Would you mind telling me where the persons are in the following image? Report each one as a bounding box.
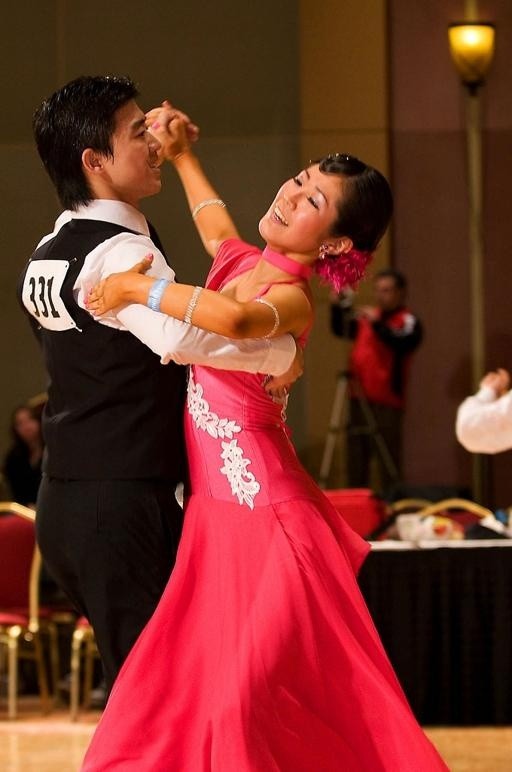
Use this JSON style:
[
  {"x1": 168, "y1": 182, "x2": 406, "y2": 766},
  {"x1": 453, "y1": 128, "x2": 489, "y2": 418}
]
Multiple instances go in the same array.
[
  {"x1": 25, "y1": 75, "x2": 302, "y2": 688},
  {"x1": 454, "y1": 361, "x2": 511, "y2": 454},
  {"x1": 2, "y1": 405, "x2": 47, "y2": 502},
  {"x1": 80, "y1": 94, "x2": 457, "y2": 772},
  {"x1": 329, "y1": 267, "x2": 423, "y2": 493}
]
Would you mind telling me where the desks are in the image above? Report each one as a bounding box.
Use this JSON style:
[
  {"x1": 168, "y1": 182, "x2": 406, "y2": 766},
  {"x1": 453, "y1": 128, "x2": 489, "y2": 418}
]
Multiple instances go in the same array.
[{"x1": 356, "y1": 548, "x2": 512, "y2": 726}]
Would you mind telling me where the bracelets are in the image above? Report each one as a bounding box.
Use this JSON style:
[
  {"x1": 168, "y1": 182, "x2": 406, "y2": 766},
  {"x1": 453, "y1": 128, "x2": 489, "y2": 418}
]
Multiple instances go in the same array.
[{"x1": 147, "y1": 276, "x2": 172, "y2": 311}]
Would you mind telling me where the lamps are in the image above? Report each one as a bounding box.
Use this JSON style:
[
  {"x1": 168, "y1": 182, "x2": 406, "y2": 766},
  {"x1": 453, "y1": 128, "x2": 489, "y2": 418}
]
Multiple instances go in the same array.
[{"x1": 448, "y1": 20, "x2": 496, "y2": 98}]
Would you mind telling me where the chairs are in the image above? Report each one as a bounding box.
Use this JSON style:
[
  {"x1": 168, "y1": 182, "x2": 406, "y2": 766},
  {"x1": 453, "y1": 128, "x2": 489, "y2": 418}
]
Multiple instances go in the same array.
[
  {"x1": 0, "y1": 502, "x2": 105, "y2": 723},
  {"x1": 387, "y1": 498, "x2": 496, "y2": 540}
]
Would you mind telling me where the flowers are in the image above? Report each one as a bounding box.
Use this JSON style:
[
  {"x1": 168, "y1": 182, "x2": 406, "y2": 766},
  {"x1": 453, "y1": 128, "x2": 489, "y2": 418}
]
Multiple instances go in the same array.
[{"x1": 317, "y1": 248, "x2": 375, "y2": 296}]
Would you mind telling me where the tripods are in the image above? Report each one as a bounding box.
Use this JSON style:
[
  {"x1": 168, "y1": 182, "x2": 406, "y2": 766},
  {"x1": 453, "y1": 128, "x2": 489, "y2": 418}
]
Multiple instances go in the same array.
[{"x1": 319, "y1": 338, "x2": 400, "y2": 489}]
